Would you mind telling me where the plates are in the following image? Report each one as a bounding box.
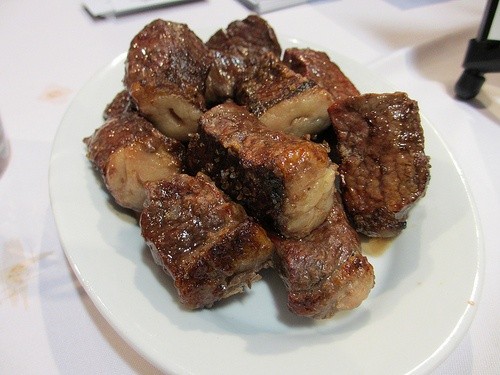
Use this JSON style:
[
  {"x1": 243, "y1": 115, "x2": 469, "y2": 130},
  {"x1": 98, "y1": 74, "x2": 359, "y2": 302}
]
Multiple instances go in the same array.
[{"x1": 46, "y1": 31, "x2": 484, "y2": 375}]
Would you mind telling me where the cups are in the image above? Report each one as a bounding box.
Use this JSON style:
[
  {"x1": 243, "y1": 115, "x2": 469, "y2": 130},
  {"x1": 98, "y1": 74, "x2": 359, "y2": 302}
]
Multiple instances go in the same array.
[{"x1": 0, "y1": 117, "x2": 13, "y2": 176}]
